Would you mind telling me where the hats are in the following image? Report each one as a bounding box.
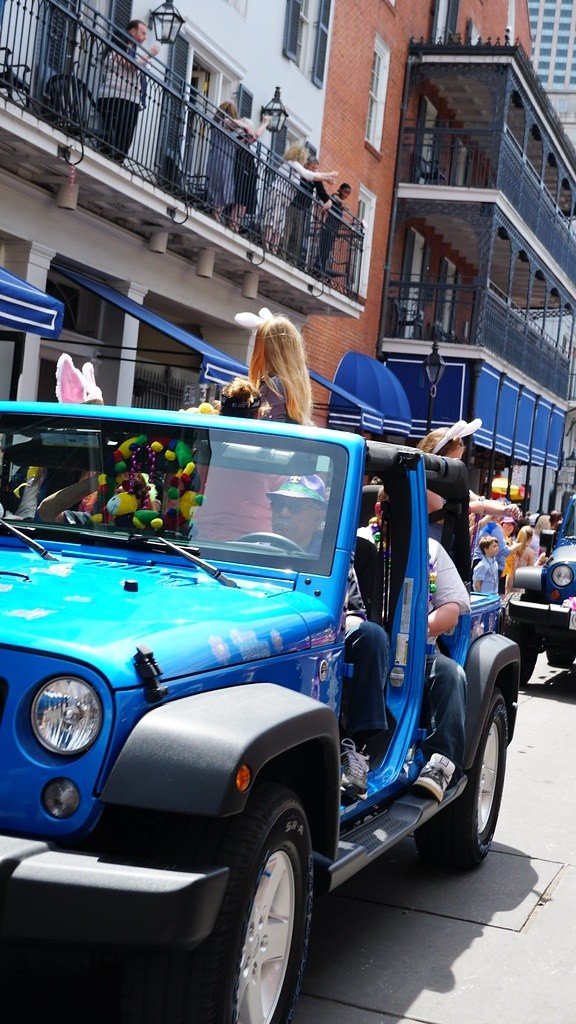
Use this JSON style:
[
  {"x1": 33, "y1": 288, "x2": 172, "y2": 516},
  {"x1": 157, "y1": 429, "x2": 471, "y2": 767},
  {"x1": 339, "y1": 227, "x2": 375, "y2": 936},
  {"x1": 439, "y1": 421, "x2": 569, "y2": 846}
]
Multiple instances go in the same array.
[
  {"x1": 500, "y1": 515, "x2": 517, "y2": 526},
  {"x1": 265, "y1": 472, "x2": 326, "y2": 503}
]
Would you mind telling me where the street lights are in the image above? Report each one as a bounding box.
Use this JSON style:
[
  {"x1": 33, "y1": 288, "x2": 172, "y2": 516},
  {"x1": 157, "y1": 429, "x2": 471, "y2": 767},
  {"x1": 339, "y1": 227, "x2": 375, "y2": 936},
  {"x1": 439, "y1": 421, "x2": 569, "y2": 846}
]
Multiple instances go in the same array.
[{"x1": 423, "y1": 338, "x2": 447, "y2": 434}]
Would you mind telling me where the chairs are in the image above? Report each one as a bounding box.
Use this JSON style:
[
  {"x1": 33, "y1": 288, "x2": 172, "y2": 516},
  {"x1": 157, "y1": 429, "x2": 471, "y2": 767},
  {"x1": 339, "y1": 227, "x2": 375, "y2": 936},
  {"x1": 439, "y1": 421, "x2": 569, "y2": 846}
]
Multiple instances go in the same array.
[
  {"x1": 166, "y1": 154, "x2": 215, "y2": 215},
  {"x1": 325, "y1": 253, "x2": 350, "y2": 285},
  {"x1": 42, "y1": 74, "x2": 115, "y2": 159},
  {"x1": 393, "y1": 297, "x2": 466, "y2": 344},
  {"x1": 225, "y1": 201, "x2": 262, "y2": 243},
  {"x1": 310, "y1": 529, "x2": 378, "y2": 621},
  {"x1": 415, "y1": 153, "x2": 448, "y2": 185},
  {"x1": 0, "y1": 47, "x2": 31, "y2": 110}
]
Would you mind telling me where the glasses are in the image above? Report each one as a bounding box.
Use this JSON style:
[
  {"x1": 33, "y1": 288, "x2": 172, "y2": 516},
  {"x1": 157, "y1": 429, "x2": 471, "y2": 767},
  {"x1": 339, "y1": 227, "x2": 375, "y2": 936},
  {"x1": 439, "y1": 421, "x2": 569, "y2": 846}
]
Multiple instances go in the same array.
[{"x1": 268, "y1": 498, "x2": 306, "y2": 514}]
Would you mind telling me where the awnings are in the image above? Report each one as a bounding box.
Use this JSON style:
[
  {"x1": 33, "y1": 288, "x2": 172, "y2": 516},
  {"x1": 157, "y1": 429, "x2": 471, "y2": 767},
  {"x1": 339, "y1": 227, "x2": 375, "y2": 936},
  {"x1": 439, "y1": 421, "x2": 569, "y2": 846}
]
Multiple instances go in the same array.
[
  {"x1": 0, "y1": 265, "x2": 62, "y2": 339},
  {"x1": 52, "y1": 263, "x2": 249, "y2": 388},
  {"x1": 326, "y1": 350, "x2": 413, "y2": 437},
  {"x1": 306, "y1": 365, "x2": 384, "y2": 433}
]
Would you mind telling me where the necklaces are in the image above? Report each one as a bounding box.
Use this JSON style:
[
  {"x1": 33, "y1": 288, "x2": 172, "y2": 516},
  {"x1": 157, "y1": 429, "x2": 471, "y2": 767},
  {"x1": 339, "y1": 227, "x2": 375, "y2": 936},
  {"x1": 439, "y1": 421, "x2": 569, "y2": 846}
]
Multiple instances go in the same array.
[
  {"x1": 369, "y1": 514, "x2": 436, "y2": 603},
  {"x1": 471, "y1": 496, "x2": 485, "y2": 557}
]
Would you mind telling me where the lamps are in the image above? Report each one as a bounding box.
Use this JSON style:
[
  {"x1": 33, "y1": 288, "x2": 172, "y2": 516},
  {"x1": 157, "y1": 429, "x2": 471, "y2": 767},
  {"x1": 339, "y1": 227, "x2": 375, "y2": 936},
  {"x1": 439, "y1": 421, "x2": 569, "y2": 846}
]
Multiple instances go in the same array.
[
  {"x1": 259, "y1": 86, "x2": 288, "y2": 133},
  {"x1": 149, "y1": 0, "x2": 185, "y2": 45}
]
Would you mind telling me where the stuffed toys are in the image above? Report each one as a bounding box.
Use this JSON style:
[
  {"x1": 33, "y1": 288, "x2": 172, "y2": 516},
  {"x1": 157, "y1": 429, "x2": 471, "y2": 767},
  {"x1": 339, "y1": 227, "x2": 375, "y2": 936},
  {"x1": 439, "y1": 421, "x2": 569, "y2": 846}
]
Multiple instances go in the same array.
[{"x1": 94, "y1": 435, "x2": 205, "y2": 532}]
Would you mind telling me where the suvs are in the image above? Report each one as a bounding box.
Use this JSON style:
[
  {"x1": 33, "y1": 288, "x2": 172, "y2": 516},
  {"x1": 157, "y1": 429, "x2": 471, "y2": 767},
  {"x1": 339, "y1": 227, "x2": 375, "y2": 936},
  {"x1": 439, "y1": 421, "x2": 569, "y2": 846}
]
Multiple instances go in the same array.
[
  {"x1": 0, "y1": 400, "x2": 524, "y2": 1024},
  {"x1": 500, "y1": 493, "x2": 576, "y2": 686}
]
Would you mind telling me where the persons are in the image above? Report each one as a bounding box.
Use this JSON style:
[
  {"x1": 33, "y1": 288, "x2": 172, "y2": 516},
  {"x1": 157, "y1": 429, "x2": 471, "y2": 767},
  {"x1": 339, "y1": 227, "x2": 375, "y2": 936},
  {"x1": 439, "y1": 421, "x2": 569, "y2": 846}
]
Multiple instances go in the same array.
[
  {"x1": 251, "y1": 476, "x2": 391, "y2": 801},
  {"x1": 96, "y1": 19, "x2": 160, "y2": 167},
  {"x1": 248, "y1": 312, "x2": 319, "y2": 427},
  {"x1": 356, "y1": 482, "x2": 470, "y2": 805},
  {"x1": 36, "y1": 472, "x2": 103, "y2": 526},
  {"x1": 417, "y1": 427, "x2": 522, "y2": 519},
  {"x1": 208, "y1": 101, "x2": 272, "y2": 234},
  {"x1": 269, "y1": 144, "x2": 351, "y2": 286},
  {"x1": 465, "y1": 488, "x2": 563, "y2": 635},
  {"x1": 8, "y1": 465, "x2": 79, "y2": 523}
]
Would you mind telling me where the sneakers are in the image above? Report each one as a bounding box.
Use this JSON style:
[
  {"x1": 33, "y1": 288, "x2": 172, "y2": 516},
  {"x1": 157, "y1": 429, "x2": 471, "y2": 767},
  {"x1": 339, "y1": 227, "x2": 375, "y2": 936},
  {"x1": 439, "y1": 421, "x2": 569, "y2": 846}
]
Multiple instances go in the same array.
[
  {"x1": 340, "y1": 736, "x2": 370, "y2": 801},
  {"x1": 412, "y1": 752, "x2": 456, "y2": 803}
]
